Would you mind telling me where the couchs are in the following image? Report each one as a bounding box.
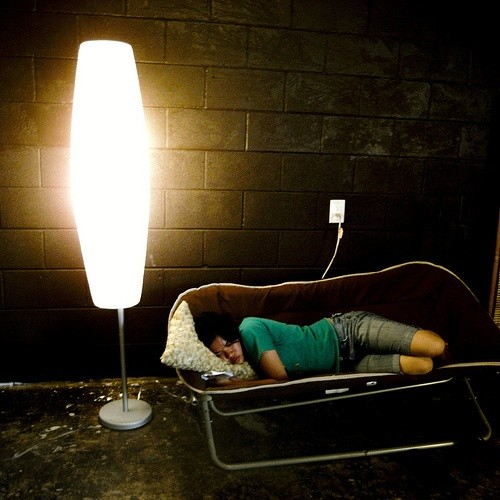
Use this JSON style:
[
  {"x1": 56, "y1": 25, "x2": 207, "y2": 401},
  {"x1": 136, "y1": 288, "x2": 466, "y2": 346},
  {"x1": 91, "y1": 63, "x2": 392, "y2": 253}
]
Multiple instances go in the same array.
[{"x1": 167, "y1": 261, "x2": 500, "y2": 472}]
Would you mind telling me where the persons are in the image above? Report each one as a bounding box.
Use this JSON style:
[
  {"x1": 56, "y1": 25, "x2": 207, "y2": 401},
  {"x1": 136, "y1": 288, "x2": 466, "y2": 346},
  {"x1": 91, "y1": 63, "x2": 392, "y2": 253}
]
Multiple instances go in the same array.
[{"x1": 200, "y1": 308, "x2": 448, "y2": 388}]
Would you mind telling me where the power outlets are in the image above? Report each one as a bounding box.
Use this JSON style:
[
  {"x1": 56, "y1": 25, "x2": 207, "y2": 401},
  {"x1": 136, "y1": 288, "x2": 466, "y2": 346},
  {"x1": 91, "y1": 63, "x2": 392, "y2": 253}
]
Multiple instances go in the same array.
[{"x1": 328, "y1": 199, "x2": 346, "y2": 224}]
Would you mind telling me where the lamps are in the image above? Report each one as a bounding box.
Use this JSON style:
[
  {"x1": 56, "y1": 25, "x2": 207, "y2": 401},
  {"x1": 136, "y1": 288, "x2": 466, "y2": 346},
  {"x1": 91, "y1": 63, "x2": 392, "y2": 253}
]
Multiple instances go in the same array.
[{"x1": 66, "y1": 38, "x2": 156, "y2": 432}]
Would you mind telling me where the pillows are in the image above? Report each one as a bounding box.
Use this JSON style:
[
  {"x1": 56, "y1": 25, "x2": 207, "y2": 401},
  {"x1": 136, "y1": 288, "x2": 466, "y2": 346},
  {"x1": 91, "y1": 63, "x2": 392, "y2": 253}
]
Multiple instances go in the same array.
[{"x1": 159, "y1": 299, "x2": 260, "y2": 381}]
